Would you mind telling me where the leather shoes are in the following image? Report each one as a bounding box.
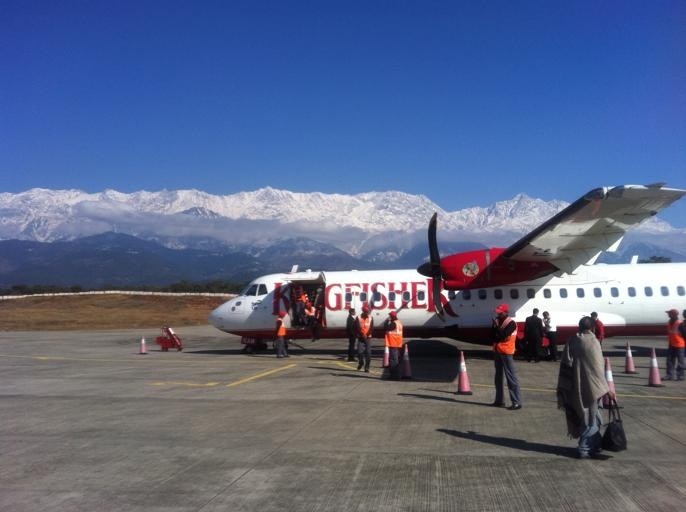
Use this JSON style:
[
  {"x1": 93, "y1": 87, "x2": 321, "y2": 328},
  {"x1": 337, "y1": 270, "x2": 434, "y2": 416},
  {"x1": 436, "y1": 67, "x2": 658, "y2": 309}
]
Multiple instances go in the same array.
[
  {"x1": 488, "y1": 402, "x2": 505, "y2": 407},
  {"x1": 508, "y1": 404, "x2": 521, "y2": 410}
]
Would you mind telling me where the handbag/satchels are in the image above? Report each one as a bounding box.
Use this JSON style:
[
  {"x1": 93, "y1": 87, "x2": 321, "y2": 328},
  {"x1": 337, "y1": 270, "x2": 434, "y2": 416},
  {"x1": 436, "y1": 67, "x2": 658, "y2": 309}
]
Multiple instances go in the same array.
[{"x1": 601, "y1": 419, "x2": 627, "y2": 451}]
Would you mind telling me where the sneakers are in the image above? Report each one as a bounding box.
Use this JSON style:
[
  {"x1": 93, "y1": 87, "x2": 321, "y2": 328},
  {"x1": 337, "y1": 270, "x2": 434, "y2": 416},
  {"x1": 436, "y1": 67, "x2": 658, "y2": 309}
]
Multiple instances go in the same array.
[
  {"x1": 357, "y1": 362, "x2": 364, "y2": 370},
  {"x1": 277, "y1": 355, "x2": 290, "y2": 359},
  {"x1": 677, "y1": 376, "x2": 686, "y2": 381},
  {"x1": 364, "y1": 366, "x2": 369, "y2": 372},
  {"x1": 663, "y1": 376, "x2": 673, "y2": 381}
]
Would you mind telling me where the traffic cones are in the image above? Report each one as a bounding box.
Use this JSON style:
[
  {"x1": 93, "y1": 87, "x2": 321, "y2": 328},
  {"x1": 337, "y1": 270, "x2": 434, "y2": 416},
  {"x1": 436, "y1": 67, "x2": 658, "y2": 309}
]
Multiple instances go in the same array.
[
  {"x1": 647, "y1": 346, "x2": 666, "y2": 386},
  {"x1": 458, "y1": 349, "x2": 474, "y2": 394},
  {"x1": 624, "y1": 341, "x2": 640, "y2": 374},
  {"x1": 137, "y1": 336, "x2": 148, "y2": 355},
  {"x1": 382, "y1": 332, "x2": 390, "y2": 368},
  {"x1": 601, "y1": 357, "x2": 624, "y2": 408},
  {"x1": 402, "y1": 342, "x2": 416, "y2": 379}
]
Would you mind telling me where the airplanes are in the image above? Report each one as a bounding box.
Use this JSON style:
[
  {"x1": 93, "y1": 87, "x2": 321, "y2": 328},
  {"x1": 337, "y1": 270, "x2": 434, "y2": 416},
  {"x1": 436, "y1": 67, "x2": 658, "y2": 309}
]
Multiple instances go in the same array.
[{"x1": 208, "y1": 182, "x2": 686, "y2": 354}]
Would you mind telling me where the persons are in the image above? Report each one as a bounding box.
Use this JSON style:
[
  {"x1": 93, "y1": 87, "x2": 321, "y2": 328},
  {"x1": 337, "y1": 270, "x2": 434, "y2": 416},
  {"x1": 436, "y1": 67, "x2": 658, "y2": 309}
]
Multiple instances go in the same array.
[
  {"x1": 556, "y1": 316, "x2": 615, "y2": 460},
  {"x1": 491, "y1": 304, "x2": 521, "y2": 410},
  {"x1": 274, "y1": 311, "x2": 288, "y2": 359},
  {"x1": 291, "y1": 285, "x2": 323, "y2": 342},
  {"x1": 524, "y1": 308, "x2": 542, "y2": 363},
  {"x1": 542, "y1": 310, "x2": 557, "y2": 362},
  {"x1": 345, "y1": 308, "x2": 357, "y2": 363},
  {"x1": 661, "y1": 309, "x2": 685, "y2": 381},
  {"x1": 353, "y1": 305, "x2": 373, "y2": 373},
  {"x1": 590, "y1": 311, "x2": 605, "y2": 344},
  {"x1": 383, "y1": 311, "x2": 402, "y2": 371}
]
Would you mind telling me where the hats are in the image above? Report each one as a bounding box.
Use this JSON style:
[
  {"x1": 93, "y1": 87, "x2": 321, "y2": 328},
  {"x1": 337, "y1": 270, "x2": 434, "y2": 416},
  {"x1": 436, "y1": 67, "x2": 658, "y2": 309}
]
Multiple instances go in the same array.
[
  {"x1": 306, "y1": 302, "x2": 311, "y2": 306},
  {"x1": 279, "y1": 310, "x2": 286, "y2": 316},
  {"x1": 362, "y1": 307, "x2": 369, "y2": 312},
  {"x1": 665, "y1": 309, "x2": 678, "y2": 314},
  {"x1": 389, "y1": 311, "x2": 396, "y2": 317},
  {"x1": 495, "y1": 304, "x2": 509, "y2": 313}
]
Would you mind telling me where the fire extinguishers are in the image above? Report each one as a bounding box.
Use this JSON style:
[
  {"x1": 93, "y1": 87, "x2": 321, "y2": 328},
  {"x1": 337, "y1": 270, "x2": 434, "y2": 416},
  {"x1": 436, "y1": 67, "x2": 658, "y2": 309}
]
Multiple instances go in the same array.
[{"x1": 162, "y1": 325, "x2": 183, "y2": 352}]
